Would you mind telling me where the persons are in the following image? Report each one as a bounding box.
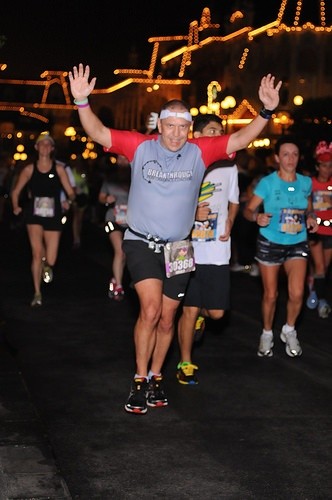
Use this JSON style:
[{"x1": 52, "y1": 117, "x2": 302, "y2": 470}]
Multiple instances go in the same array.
[
  {"x1": 68, "y1": 63, "x2": 282, "y2": 413},
  {"x1": 176, "y1": 114, "x2": 239, "y2": 384},
  {"x1": 99, "y1": 155, "x2": 132, "y2": 300},
  {"x1": 307, "y1": 141, "x2": 332, "y2": 317},
  {"x1": 11, "y1": 134, "x2": 89, "y2": 306},
  {"x1": 243, "y1": 138, "x2": 318, "y2": 358}
]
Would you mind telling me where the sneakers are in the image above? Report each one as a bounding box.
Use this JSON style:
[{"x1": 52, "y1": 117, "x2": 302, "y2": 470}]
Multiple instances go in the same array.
[
  {"x1": 176, "y1": 359, "x2": 199, "y2": 385},
  {"x1": 318, "y1": 299, "x2": 332, "y2": 318},
  {"x1": 194, "y1": 312, "x2": 205, "y2": 345},
  {"x1": 257, "y1": 329, "x2": 274, "y2": 356},
  {"x1": 109, "y1": 278, "x2": 125, "y2": 300},
  {"x1": 124, "y1": 374, "x2": 148, "y2": 414},
  {"x1": 280, "y1": 324, "x2": 302, "y2": 357},
  {"x1": 30, "y1": 293, "x2": 42, "y2": 306},
  {"x1": 41, "y1": 256, "x2": 53, "y2": 283},
  {"x1": 146, "y1": 371, "x2": 168, "y2": 408},
  {"x1": 306, "y1": 277, "x2": 318, "y2": 309}
]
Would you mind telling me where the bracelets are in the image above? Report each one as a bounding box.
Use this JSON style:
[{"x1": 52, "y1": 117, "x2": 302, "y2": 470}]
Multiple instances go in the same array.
[
  {"x1": 74, "y1": 98, "x2": 89, "y2": 108},
  {"x1": 260, "y1": 106, "x2": 275, "y2": 119}
]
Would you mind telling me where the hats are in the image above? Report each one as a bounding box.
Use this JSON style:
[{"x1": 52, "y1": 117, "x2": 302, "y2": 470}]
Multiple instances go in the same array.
[
  {"x1": 34, "y1": 135, "x2": 55, "y2": 151},
  {"x1": 315, "y1": 152, "x2": 332, "y2": 163}
]
